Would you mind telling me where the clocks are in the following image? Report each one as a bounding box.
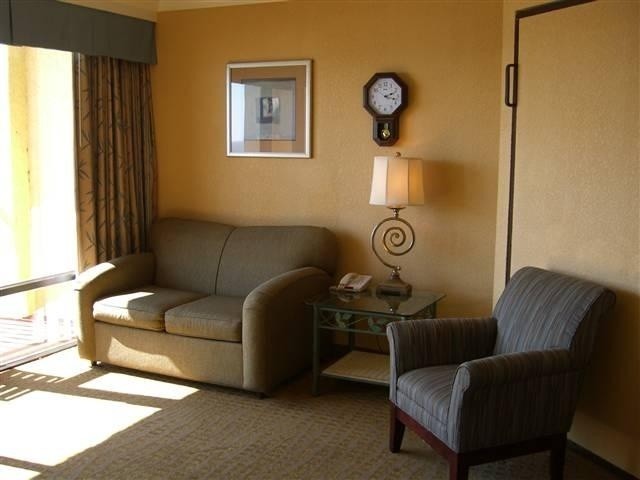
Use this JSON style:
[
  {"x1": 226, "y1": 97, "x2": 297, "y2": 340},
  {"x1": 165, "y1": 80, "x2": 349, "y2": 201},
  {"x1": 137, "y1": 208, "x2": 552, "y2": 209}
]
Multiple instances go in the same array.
[{"x1": 362, "y1": 71, "x2": 408, "y2": 147}]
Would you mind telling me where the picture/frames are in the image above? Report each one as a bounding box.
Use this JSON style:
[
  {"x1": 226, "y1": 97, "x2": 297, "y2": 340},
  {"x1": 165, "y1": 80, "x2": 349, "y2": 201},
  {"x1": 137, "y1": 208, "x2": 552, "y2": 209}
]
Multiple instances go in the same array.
[{"x1": 224, "y1": 58, "x2": 313, "y2": 159}]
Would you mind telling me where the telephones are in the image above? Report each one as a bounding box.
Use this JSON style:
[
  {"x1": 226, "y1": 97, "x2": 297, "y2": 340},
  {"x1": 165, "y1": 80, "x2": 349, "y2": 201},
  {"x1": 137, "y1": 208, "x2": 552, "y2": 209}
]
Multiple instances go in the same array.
[{"x1": 338, "y1": 272, "x2": 373, "y2": 293}]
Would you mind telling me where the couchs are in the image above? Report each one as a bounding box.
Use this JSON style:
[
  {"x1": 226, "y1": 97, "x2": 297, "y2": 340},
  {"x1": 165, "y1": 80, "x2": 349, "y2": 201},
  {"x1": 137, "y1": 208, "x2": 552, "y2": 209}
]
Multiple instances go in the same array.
[{"x1": 71, "y1": 216, "x2": 342, "y2": 402}]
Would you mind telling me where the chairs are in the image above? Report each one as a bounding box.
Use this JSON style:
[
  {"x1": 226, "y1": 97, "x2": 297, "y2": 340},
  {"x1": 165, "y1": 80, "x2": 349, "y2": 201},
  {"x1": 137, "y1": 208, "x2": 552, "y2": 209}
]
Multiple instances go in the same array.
[{"x1": 384, "y1": 266, "x2": 617, "y2": 478}]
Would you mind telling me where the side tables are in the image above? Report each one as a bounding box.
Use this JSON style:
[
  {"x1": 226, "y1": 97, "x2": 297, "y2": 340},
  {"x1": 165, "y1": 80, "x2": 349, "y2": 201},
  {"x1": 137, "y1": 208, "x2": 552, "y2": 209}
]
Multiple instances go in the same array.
[{"x1": 307, "y1": 286, "x2": 448, "y2": 402}]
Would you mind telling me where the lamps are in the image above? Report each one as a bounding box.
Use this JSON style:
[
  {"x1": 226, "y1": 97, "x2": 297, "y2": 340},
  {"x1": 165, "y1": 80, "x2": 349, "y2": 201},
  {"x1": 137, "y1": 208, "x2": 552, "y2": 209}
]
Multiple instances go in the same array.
[{"x1": 368, "y1": 151, "x2": 428, "y2": 296}]
